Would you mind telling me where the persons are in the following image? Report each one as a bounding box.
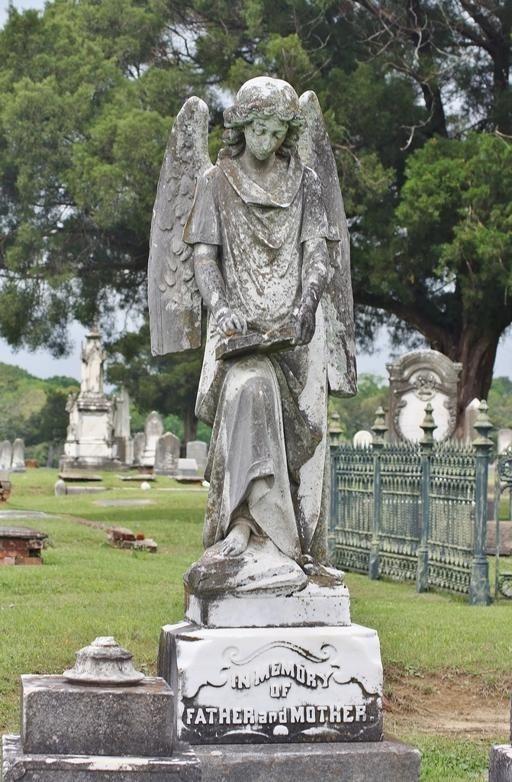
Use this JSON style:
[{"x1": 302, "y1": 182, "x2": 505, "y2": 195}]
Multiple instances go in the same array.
[{"x1": 182, "y1": 75, "x2": 332, "y2": 597}]
